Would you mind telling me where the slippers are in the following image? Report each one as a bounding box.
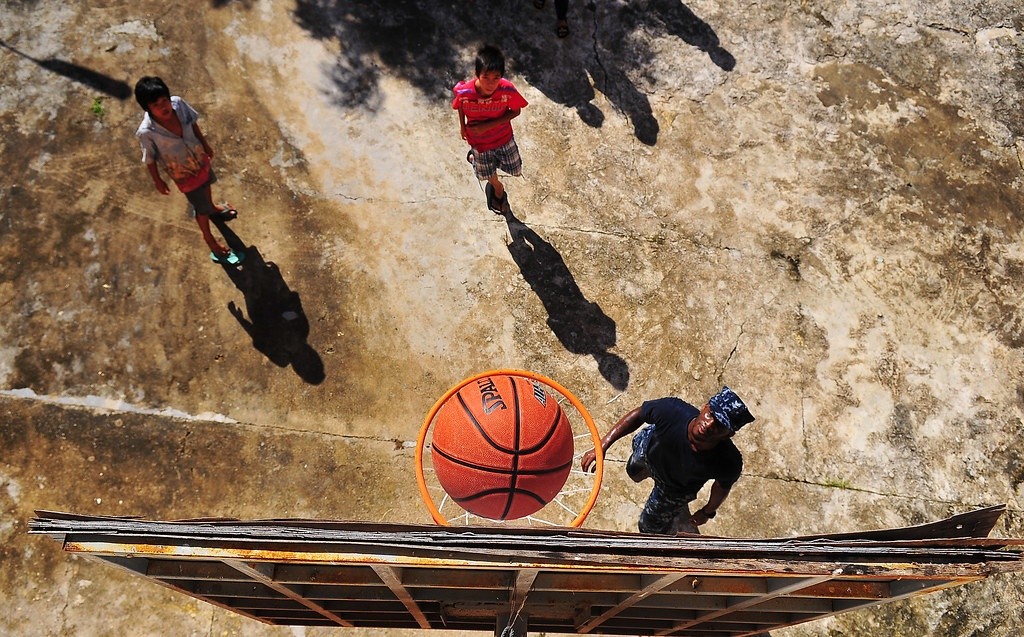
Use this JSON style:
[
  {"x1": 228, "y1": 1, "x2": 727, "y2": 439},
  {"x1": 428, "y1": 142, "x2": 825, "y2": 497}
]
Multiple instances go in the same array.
[
  {"x1": 491, "y1": 192, "x2": 507, "y2": 216},
  {"x1": 210, "y1": 201, "x2": 237, "y2": 218},
  {"x1": 466, "y1": 150, "x2": 475, "y2": 164},
  {"x1": 210, "y1": 249, "x2": 246, "y2": 266}
]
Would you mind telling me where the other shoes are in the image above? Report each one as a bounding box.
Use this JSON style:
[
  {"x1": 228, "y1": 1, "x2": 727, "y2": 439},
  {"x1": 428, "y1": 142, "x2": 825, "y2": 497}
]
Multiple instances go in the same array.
[
  {"x1": 557, "y1": 25, "x2": 569, "y2": 37},
  {"x1": 534, "y1": 0, "x2": 545, "y2": 9}
]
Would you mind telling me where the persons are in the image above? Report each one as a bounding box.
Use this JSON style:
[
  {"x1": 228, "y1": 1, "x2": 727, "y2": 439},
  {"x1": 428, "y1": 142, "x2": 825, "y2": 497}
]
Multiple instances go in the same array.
[
  {"x1": 582, "y1": 385, "x2": 755, "y2": 534},
  {"x1": 451, "y1": 47, "x2": 528, "y2": 214},
  {"x1": 135, "y1": 75, "x2": 244, "y2": 265}
]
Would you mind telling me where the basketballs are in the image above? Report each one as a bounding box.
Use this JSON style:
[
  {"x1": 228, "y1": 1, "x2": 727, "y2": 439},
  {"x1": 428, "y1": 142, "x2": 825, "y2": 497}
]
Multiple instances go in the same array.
[{"x1": 432, "y1": 375, "x2": 574, "y2": 520}]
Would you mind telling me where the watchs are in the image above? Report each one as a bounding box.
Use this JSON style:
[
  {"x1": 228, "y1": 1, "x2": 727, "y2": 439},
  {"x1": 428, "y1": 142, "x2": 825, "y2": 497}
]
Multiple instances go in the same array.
[{"x1": 702, "y1": 507, "x2": 716, "y2": 518}]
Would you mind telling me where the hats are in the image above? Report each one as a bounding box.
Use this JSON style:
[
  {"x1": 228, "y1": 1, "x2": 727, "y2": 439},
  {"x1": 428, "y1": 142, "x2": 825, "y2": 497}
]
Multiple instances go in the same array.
[{"x1": 709, "y1": 386, "x2": 755, "y2": 433}]
